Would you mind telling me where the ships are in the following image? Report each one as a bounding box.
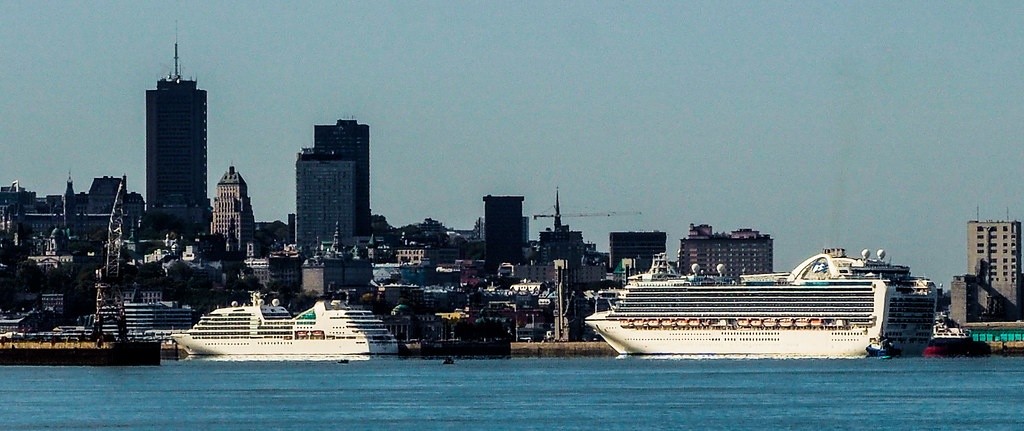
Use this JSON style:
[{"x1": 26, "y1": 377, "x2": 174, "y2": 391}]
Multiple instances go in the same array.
[{"x1": 584, "y1": 252, "x2": 936, "y2": 359}]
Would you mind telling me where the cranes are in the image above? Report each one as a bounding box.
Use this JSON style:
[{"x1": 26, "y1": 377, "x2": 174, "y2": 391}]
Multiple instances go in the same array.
[{"x1": 533, "y1": 186, "x2": 642, "y2": 231}]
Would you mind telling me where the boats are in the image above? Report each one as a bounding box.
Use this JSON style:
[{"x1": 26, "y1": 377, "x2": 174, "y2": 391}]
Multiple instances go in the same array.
[
  {"x1": 338, "y1": 359, "x2": 349, "y2": 364},
  {"x1": 932, "y1": 311, "x2": 972, "y2": 341},
  {"x1": 171, "y1": 291, "x2": 399, "y2": 356},
  {"x1": 443, "y1": 355, "x2": 454, "y2": 364},
  {"x1": 864, "y1": 345, "x2": 900, "y2": 356},
  {"x1": 0, "y1": 176, "x2": 162, "y2": 366}
]
